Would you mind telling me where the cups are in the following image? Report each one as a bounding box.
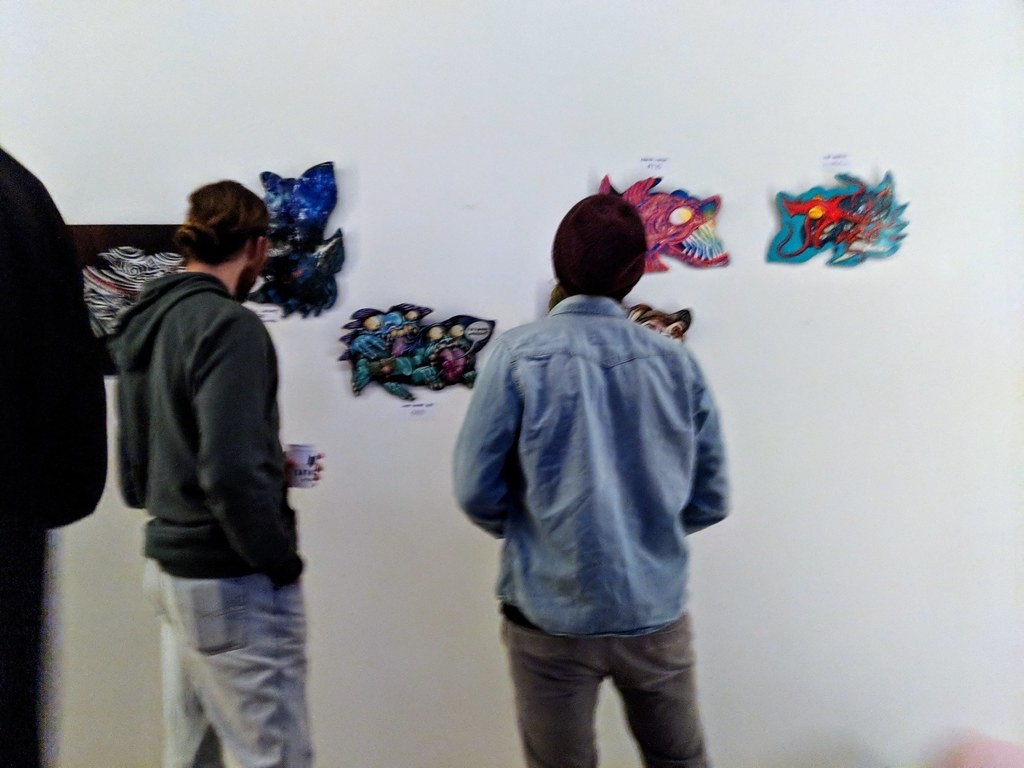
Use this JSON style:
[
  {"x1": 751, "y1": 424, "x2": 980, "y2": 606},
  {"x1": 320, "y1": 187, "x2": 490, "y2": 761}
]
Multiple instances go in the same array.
[{"x1": 285, "y1": 443, "x2": 319, "y2": 489}]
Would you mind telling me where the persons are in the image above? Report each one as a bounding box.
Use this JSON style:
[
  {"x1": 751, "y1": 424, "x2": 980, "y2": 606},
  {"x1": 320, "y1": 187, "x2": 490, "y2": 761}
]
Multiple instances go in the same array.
[
  {"x1": 450, "y1": 194, "x2": 736, "y2": 767},
  {"x1": 110, "y1": 180, "x2": 326, "y2": 767},
  {"x1": 0, "y1": 147, "x2": 108, "y2": 768}
]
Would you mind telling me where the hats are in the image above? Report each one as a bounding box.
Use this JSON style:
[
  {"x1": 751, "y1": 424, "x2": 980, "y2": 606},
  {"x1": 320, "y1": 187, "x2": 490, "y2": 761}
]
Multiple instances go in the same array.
[{"x1": 554, "y1": 193, "x2": 646, "y2": 297}]
